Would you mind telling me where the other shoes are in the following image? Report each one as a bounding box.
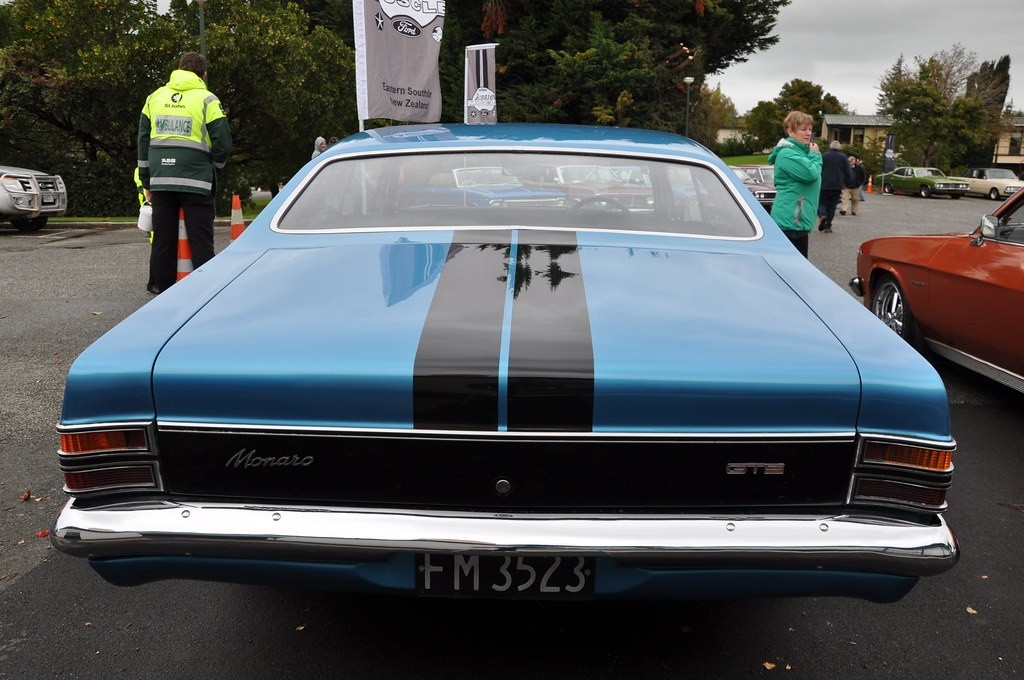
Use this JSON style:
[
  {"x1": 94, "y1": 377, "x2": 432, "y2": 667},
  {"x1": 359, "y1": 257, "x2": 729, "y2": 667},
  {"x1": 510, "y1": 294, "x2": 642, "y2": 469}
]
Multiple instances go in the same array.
[
  {"x1": 853, "y1": 212, "x2": 856, "y2": 215},
  {"x1": 841, "y1": 210, "x2": 846, "y2": 215},
  {"x1": 818, "y1": 216, "x2": 832, "y2": 233}
]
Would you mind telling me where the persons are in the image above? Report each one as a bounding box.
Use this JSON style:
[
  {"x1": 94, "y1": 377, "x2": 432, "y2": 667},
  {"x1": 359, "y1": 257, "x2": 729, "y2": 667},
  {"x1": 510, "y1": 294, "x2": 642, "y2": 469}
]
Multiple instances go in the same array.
[
  {"x1": 311, "y1": 136, "x2": 339, "y2": 160},
  {"x1": 819, "y1": 140, "x2": 851, "y2": 233},
  {"x1": 134, "y1": 52, "x2": 234, "y2": 296},
  {"x1": 769, "y1": 111, "x2": 822, "y2": 260},
  {"x1": 839, "y1": 155, "x2": 868, "y2": 214}
]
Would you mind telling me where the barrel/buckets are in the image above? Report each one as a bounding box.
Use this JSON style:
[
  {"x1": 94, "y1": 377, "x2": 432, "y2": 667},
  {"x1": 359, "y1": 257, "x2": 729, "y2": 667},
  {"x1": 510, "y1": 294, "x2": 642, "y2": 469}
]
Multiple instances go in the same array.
[{"x1": 137, "y1": 200, "x2": 153, "y2": 231}]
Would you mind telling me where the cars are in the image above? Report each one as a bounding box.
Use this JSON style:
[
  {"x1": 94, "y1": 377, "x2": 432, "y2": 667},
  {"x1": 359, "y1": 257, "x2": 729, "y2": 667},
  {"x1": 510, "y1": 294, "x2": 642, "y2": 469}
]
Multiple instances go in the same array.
[
  {"x1": 519, "y1": 165, "x2": 654, "y2": 211},
  {"x1": 948, "y1": 167, "x2": 1024, "y2": 201},
  {"x1": 849, "y1": 186, "x2": 1024, "y2": 394},
  {"x1": 410, "y1": 166, "x2": 566, "y2": 208},
  {"x1": 875, "y1": 167, "x2": 970, "y2": 200},
  {"x1": 726, "y1": 167, "x2": 777, "y2": 204},
  {"x1": 57, "y1": 121, "x2": 960, "y2": 604},
  {"x1": 0, "y1": 166, "x2": 67, "y2": 231},
  {"x1": 741, "y1": 167, "x2": 775, "y2": 185}
]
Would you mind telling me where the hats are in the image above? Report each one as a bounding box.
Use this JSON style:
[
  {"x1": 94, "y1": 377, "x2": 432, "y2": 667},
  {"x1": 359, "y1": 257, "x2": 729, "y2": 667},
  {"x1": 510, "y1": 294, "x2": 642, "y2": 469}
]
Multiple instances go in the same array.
[
  {"x1": 330, "y1": 137, "x2": 339, "y2": 144},
  {"x1": 848, "y1": 156, "x2": 855, "y2": 162}
]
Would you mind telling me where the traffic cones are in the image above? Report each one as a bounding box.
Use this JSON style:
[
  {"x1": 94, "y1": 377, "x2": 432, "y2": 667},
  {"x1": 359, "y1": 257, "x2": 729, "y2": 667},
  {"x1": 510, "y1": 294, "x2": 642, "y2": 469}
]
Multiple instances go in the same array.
[
  {"x1": 229, "y1": 191, "x2": 245, "y2": 244},
  {"x1": 175, "y1": 207, "x2": 193, "y2": 281},
  {"x1": 866, "y1": 173, "x2": 873, "y2": 192}
]
